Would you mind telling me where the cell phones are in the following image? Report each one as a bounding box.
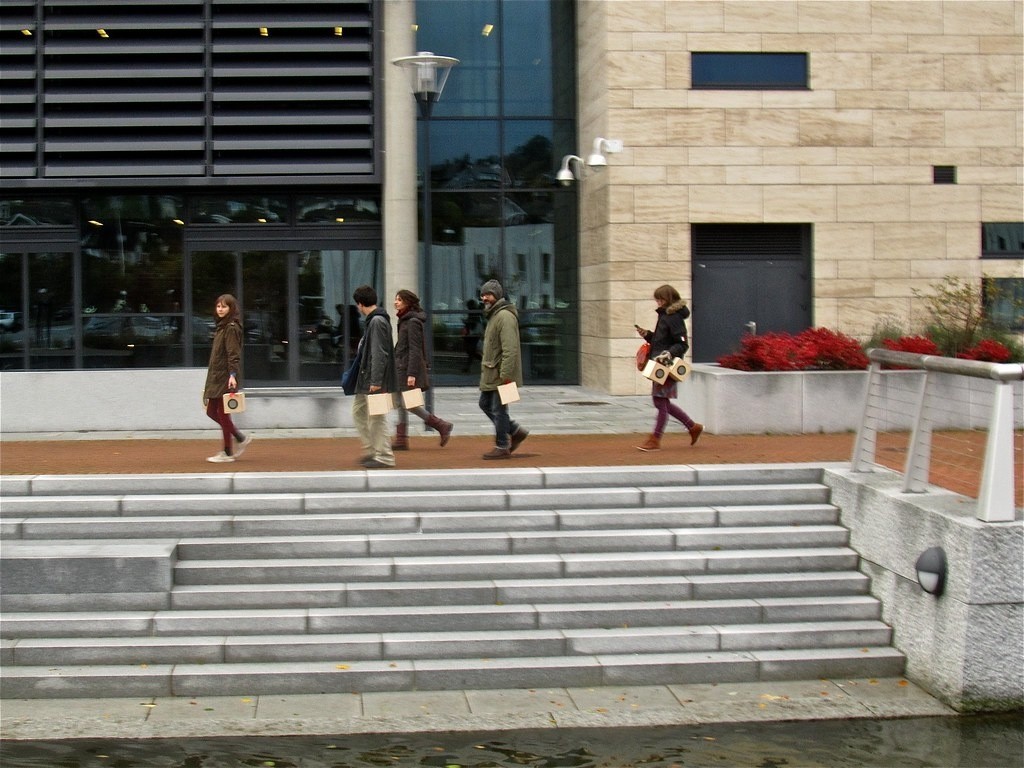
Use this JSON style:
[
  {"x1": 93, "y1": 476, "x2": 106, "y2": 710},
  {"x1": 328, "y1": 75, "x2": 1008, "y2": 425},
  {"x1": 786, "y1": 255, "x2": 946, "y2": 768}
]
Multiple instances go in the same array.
[{"x1": 634, "y1": 325, "x2": 644, "y2": 334}]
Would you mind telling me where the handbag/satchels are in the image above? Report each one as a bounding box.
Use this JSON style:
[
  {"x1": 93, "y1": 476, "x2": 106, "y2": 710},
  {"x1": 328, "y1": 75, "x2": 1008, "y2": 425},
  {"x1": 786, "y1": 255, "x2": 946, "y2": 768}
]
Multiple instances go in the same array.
[
  {"x1": 637, "y1": 341, "x2": 651, "y2": 372},
  {"x1": 340, "y1": 335, "x2": 366, "y2": 395}
]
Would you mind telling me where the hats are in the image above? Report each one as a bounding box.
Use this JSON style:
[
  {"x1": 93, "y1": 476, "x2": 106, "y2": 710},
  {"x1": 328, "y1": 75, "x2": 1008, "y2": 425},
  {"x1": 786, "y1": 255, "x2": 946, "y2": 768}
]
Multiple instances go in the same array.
[{"x1": 480, "y1": 279, "x2": 503, "y2": 300}]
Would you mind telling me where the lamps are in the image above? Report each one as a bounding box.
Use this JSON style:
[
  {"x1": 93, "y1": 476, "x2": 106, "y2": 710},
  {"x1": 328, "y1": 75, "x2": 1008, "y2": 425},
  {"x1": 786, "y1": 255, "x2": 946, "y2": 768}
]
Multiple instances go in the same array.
[
  {"x1": 541, "y1": 166, "x2": 556, "y2": 185},
  {"x1": 587, "y1": 138, "x2": 624, "y2": 172},
  {"x1": 558, "y1": 154, "x2": 587, "y2": 187}
]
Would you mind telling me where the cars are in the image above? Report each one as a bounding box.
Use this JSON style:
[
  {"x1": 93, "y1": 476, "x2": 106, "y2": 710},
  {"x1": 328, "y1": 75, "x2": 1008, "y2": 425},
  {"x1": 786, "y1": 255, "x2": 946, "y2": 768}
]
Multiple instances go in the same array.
[
  {"x1": 0, "y1": 312, "x2": 22, "y2": 333},
  {"x1": 70, "y1": 312, "x2": 164, "y2": 345}
]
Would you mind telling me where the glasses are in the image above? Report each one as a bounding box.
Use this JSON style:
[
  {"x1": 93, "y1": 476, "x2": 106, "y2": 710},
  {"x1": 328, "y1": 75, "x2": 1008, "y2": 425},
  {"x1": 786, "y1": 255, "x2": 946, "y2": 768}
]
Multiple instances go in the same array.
[{"x1": 480, "y1": 293, "x2": 492, "y2": 299}]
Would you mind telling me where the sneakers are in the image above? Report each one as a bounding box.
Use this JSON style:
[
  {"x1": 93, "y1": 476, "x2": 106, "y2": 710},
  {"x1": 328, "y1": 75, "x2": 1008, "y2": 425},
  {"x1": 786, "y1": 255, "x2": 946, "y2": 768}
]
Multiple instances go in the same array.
[
  {"x1": 635, "y1": 435, "x2": 661, "y2": 451},
  {"x1": 206, "y1": 450, "x2": 235, "y2": 463},
  {"x1": 688, "y1": 423, "x2": 705, "y2": 446},
  {"x1": 235, "y1": 436, "x2": 253, "y2": 457}
]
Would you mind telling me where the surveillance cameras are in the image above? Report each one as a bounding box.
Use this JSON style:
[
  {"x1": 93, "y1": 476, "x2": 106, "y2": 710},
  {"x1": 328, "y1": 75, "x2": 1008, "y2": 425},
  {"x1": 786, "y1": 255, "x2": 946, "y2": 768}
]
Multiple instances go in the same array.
[
  {"x1": 587, "y1": 155, "x2": 608, "y2": 173},
  {"x1": 555, "y1": 168, "x2": 574, "y2": 186}
]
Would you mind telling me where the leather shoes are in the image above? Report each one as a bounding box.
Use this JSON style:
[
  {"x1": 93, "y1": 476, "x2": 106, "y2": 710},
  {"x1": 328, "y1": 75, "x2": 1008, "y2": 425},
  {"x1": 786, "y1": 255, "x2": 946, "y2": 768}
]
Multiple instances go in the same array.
[
  {"x1": 509, "y1": 426, "x2": 529, "y2": 453},
  {"x1": 481, "y1": 446, "x2": 511, "y2": 460}
]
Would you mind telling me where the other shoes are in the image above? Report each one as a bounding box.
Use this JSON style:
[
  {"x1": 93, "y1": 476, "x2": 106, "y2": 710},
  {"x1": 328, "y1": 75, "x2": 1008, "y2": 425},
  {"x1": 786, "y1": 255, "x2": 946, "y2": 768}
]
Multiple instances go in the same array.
[{"x1": 356, "y1": 455, "x2": 394, "y2": 468}]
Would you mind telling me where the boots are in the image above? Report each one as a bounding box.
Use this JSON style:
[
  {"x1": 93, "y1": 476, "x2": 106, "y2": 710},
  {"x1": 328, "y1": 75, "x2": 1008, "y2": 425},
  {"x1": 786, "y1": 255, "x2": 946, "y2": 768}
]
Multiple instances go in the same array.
[
  {"x1": 425, "y1": 412, "x2": 453, "y2": 447},
  {"x1": 392, "y1": 423, "x2": 409, "y2": 450}
]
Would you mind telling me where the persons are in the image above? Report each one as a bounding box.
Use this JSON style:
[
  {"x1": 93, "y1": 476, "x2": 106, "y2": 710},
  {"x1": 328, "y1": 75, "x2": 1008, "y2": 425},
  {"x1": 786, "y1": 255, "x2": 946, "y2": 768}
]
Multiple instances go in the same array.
[
  {"x1": 334, "y1": 303, "x2": 364, "y2": 347},
  {"x1": 460, "y1": 293, "x2": 557, "y2": 374},
  {"x1": 351, "y1": 285, "x2": 396, "y2": 467},
  {"x1": 204, "y1": 294, "x2": 253, "y2": 462},
  {"x1": 392, "y1": 290, "x2": 453, "y2": 451},
  {"x1": 113, "y1": 290, "x2": 131, "y2": 312},
  {"x1": 635, "y1": 285, "x2": 705, "y2": 452},
  {"x1": 479, "y1": 280, "x2": 530, "y2": 460},
  {"x1": 36, "y1": 289, "x2": 54, "y2": 347}
]
